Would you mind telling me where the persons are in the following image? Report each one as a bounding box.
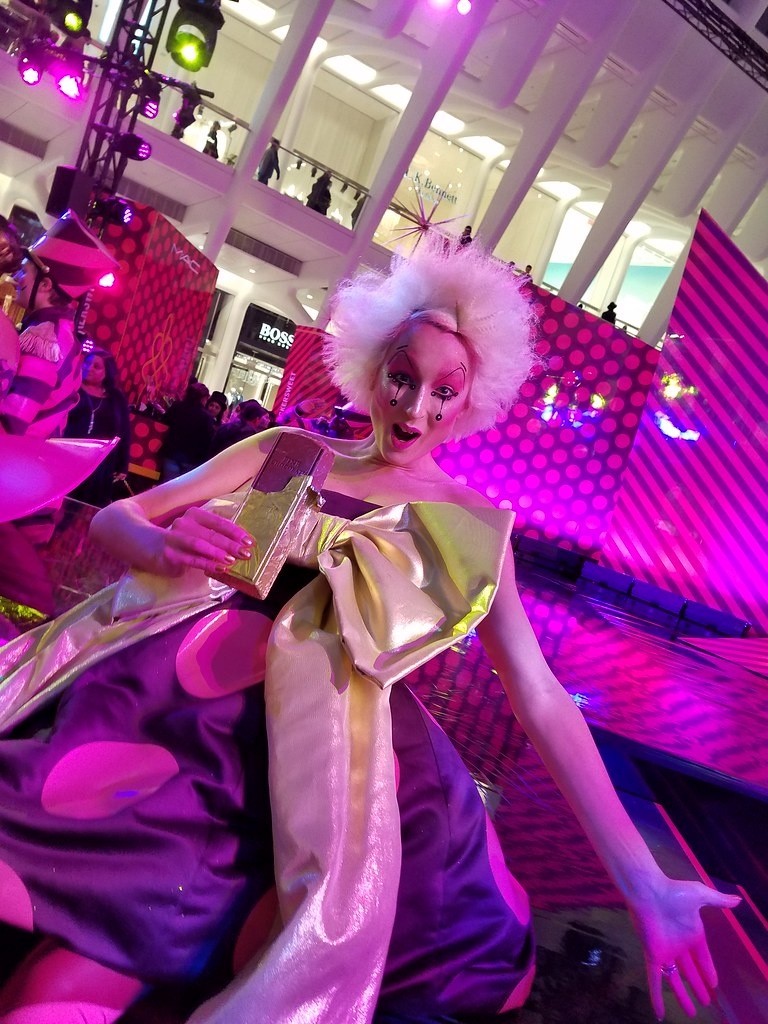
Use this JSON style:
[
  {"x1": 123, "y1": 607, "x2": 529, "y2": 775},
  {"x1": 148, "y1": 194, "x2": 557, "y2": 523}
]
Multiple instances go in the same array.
[
  {"x1": 0, "y1": 238, "x2": 742, "y2": 1024},
  {"x1": 171, "y1": 82, "x2": 203, "y2": 140},
  {"x1": 157, "y1": 383, "x2": 283, "y2": 485},
  {"x1": 459, "y1": 226, "x2": 472, "y2": 244},
  {"x1": 306, "y1": 171, "x2": 332, "y2": 216},
  {"x1": 0, "y1": 207, "x2": 122, "y2": 556},
  {"x1": 602, "y1": 302, "x2": 617, "y2": 325},
  {"x1": 64, "y1": 350, "x2": 129, "y2": 502},
  {"x1": 519, "y1": 265, "x2": 533, "y2": 283},
  {"x1": 258, "y1": 140, "x2": 280, "y2": 186},
  {"x1": 202, "y1": 121, "x2": 221, "y2": 160}
]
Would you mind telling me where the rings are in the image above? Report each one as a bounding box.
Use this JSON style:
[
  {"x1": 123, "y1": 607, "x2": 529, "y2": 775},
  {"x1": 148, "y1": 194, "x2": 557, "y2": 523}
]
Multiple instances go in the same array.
[{"x1": 662, "y1": 964, "x2": 677, "y2": 975}]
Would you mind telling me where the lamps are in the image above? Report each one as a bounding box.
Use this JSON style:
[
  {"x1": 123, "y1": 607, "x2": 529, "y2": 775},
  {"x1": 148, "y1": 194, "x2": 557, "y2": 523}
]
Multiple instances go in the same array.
[
  {"x1": 16, "y1": 0, "x2": 97, "y2": 97},
  {"x1": 166, "y1": 0, "x2": 226, "y2": 72},
  {"x1": 134, "y1": 77, "x2": 160, "y2": 118},
  {"x1": 92, "y1": 198, "x2": 133, "y2": 226},
  {"x1": 106, "y1": 130, "x2": 153, "y2": 161}
]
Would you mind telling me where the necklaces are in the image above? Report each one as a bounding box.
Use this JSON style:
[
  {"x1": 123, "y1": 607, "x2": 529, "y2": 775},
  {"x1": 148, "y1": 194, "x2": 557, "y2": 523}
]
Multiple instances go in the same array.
[{"x1": 84, "y1": 386, "x2": 106, "y2": 434}]
